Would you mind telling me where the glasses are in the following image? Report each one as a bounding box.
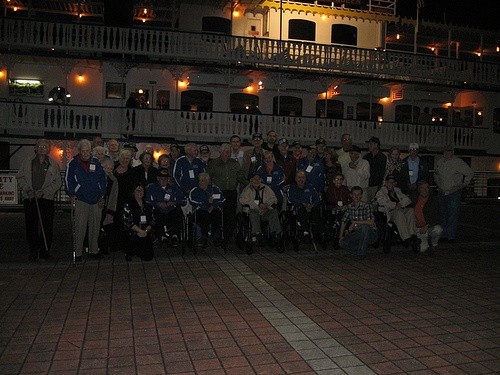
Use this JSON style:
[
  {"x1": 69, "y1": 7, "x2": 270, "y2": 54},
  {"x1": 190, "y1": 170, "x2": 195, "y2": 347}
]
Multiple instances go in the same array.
[
  {"x1": 37, "y1": 144, "x2": 48, "y2": 148},
  {"x1": 253, "y1": 138, "x2": 261, "y2": 141},
  {"x1": 335, "y1": 177, "x2": 343, "y2": 181},
  {"x1": 344, "y1": 138, "x2": 352, "y2": 141}
]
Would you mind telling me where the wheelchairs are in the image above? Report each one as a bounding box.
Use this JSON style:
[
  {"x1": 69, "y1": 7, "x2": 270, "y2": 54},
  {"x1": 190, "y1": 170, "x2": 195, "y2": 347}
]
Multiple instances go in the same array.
[{"x1": 97, "y1": 210, "x2": 422, "y2": 261}]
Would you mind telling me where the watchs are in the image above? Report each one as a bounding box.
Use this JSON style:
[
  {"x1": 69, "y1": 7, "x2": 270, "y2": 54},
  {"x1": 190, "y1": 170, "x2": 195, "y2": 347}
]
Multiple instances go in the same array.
[
  {"x1": 353, "y1": 221, "x2": 359, "y2": 227},
  {"x1": 144, "y1": 229, "x2": 149, "y2": 233}
]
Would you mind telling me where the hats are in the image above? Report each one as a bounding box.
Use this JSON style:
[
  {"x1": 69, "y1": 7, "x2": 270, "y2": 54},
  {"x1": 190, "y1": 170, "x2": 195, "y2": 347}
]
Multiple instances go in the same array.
[
  {"x1": 139, "y1": 151, "x2": 154, "y2": 163},
  {"x1": 252, "y1": 134, "x2": 262, "y2": 137},
  {"x1": 409, "y1": 143, "x2": 419, "y2": 151},
  {"x1": 292, "y1": 141, "x2": 302, "y2": 146},
  {"x1": 278, "y1": 138, "x2": 288, "y2": 144},
  {"x1": 123, "y1": 142, "x2": 138, "y2": 152},
  {"x1": 385, "y1": 174, "x2": 396, "y2": 180},
  {"x1": 364, "y1": 135, "x2": 380, "y2": 143},
  {"x1": 315, "y1": 138, "x2": 326, "y2": 146},
  {"x1": 199, "y1": 144, "x2": 209, "y2": 152},
  {"x1": 248, "y1": 172, "x2": 262, "y2": 180},
  {"x1": 344, "y1": 144, "x2": 362, "y2": 153},
  {"x1": 441, "y1": 143, "x2": 454, "y2": 152}
]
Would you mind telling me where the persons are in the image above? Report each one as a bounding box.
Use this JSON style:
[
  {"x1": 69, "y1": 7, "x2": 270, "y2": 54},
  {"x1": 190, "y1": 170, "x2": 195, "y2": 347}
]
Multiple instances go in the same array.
[
  {"x1": 63, "y1": 138, "x2": 108, "y2": 262},
  {"x1": 17, "y1": 139, "x2": 62, "y2": 262},
  {"x1": 83, "y1": 136, "x2": 445, "y2": 264},
  {"x1": 235, "y1": 105, "x2": 249, "y2": 122},
  {"x1": 188, "y1": 173, "x2": 226, "y2": 247},
  {"x1": 432, "y1": 143, "x2": 475, "y2": 247},
  {"x1": 125, "y1": 92, "x2": 148, "y2": 128},
  {"x1": 335, "y1": 185, "x2": 379, "y2": 262},
  {"x1": 250, "y1": 105, "x2": 262, "y2": 130},
  {"x1": 374, "y1": 174, "x2": 422, "y2": 248},
  {"x1": 239, "y1": 173, "x2": 286, "y2": 246}
]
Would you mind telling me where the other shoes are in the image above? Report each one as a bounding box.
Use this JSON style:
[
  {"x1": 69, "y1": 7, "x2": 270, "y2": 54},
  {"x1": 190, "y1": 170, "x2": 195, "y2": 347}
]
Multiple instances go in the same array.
[
  {"x1": 73, "y1": 256, "x2": 82, "y2": 264},
  {"x1": 124, "y1": 252, "x2": 133, "y2": 261},
  {"x1": 86, "y1": 252, "x2": 104, "y2": 259},
  {"x1": 405, "y1": 235, "x2": 421, "y2": 249},
  {"x1": 195, "y1": 237, "x2": 224, "y2": 248},
  {"x1": 29, "y1": 253, "x2": 53, "y2": 263},
  {"x1": 252, "y1": 234, "x2": 282, "y2": 247},
  {"x1": 160, "y1": 236, "x2": 177, "y2": 248}
]
